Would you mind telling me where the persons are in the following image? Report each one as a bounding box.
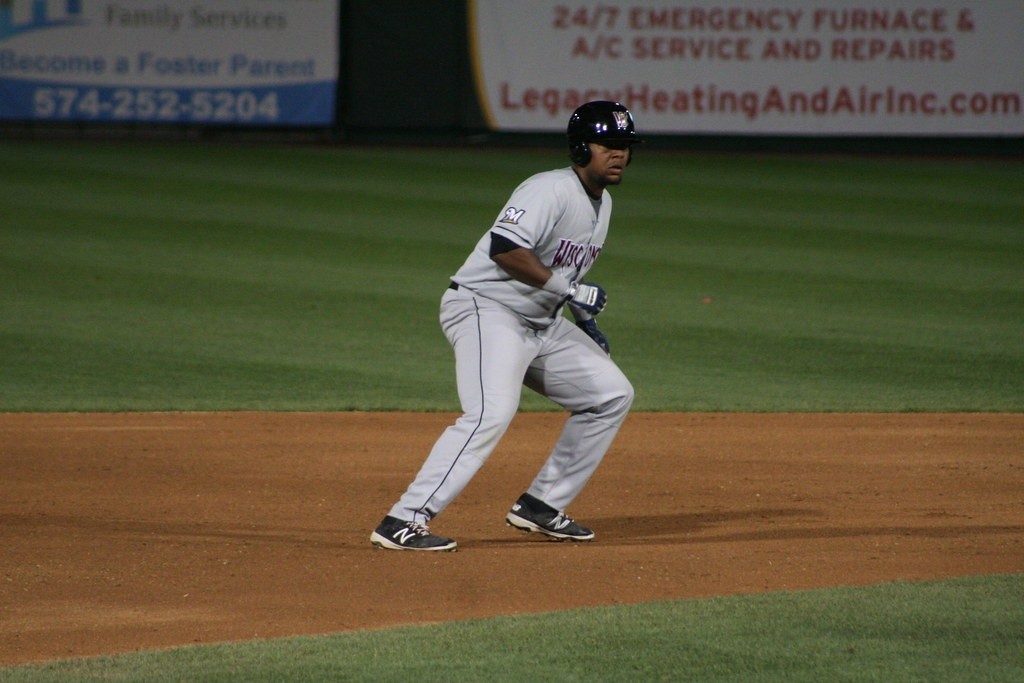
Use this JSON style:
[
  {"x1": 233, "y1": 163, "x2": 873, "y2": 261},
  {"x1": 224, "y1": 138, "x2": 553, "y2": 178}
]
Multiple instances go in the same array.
[{"x1": 369, "y1": 100, "x2": 637, "y2": 550}]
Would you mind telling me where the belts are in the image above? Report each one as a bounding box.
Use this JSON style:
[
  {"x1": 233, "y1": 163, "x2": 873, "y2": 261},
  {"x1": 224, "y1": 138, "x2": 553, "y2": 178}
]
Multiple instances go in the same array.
[{"x1": 448, "y1": 282, "x2": 460, "y2": 291}]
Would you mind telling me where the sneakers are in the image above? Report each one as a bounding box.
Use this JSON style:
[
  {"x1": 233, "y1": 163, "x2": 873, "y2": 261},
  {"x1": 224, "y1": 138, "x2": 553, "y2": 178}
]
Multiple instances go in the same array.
[
  {"x1": 369, "y1": 515, "x2": 458, "y2": 552},
  {"x1": 504, "y1": 492, "x2": 595, "y2": 542}
]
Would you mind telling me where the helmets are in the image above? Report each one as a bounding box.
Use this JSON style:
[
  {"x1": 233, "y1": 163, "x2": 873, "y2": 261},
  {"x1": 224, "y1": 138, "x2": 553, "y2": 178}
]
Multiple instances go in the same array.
[{"x1": 566, "y1": 100, "x2": 640, "y2": 168}]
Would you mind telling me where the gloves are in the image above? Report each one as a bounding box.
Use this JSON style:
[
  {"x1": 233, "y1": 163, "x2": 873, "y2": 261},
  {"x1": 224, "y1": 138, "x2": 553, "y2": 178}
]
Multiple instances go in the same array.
[
  {"x1": 575, "y1": 318, "x2": 611, "y2": 359},
  {"x1": 564, "y1": 279, "x2": 607, "y2": 315}
]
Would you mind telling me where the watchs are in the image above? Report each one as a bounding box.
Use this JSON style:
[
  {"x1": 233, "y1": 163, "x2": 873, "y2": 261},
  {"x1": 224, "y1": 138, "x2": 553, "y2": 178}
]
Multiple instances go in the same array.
[{"x1": 566, "y1": 281, "x2": 579, "y2": 302}]
